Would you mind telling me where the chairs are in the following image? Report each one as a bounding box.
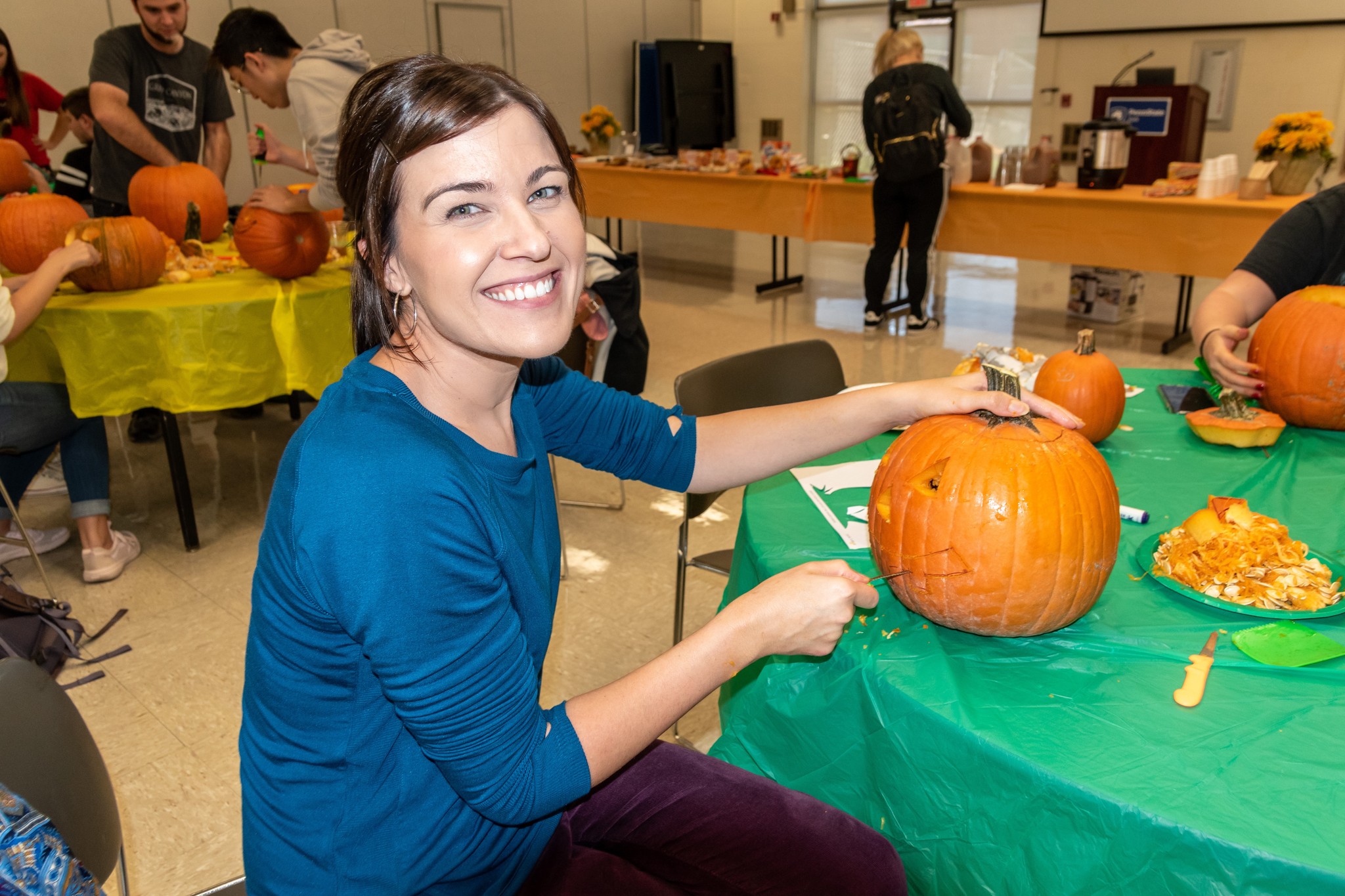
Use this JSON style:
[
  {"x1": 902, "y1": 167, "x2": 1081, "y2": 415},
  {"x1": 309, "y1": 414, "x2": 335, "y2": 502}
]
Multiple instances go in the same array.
[
  {"x1": 671, "y1": 337, "x2": 850, "y2": 645},
  {"x1": 0, "y1": 657, "x2": 248, "y2": 896}
]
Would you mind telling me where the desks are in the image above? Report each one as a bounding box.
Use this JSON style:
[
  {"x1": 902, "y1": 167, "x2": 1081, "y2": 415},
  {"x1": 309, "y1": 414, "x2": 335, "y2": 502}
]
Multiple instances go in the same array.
[{"x1": 568, "y1": 149, "x2": 1318, "y2": 357}]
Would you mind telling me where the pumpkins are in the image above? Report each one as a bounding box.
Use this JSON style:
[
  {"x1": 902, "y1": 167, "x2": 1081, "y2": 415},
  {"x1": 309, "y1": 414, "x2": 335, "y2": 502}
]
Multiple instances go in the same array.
[{"x1": 1031, "y1": 328, "x2": 1127, "y2": 449}]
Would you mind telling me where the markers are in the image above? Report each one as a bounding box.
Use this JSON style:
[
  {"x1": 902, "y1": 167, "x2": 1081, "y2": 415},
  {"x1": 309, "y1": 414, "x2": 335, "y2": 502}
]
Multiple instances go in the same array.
[{"x1": 1118, "y1": 504, "x2": 1151, "y2": 524}]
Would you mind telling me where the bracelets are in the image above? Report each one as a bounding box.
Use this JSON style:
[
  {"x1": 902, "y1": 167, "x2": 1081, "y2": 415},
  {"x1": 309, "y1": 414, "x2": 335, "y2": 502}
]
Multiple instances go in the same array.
[{"x1": 1200, "y1": 328, "x2": 1220, "y2": 360}]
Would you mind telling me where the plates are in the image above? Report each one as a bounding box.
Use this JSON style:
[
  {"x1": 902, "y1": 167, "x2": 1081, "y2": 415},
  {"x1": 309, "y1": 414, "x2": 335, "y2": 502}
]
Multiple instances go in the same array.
[{"x1": 1134, "y1": 525, "x2": 1344, "y2": 619}]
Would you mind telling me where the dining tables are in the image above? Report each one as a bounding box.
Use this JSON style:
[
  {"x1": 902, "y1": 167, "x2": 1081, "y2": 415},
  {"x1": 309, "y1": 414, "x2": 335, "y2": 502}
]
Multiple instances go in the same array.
[
  {"x1": 699, "y1": 367, "x2": 1342, "y2": 896},
  {"x1": 2, "y1": 226, "x2": 361, "y2": 548}
]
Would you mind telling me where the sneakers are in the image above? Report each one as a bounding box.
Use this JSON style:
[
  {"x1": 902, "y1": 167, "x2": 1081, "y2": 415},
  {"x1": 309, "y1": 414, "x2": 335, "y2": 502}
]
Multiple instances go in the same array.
[
  {"x1": 906, "y1": 315, "x2": 939, "y2": 336},
  {"x1": 81, "y1": 520, "x2": 141, "y2": 583},
  {"x1": 0, "y1": 523, "x2": 70, "y2": 564},
  {"x1": 864, "y1": 306, "x2": 884, "y2": 331},
  {"x1": 21, "y1": 468, "x2": 69, "y2": 496}
]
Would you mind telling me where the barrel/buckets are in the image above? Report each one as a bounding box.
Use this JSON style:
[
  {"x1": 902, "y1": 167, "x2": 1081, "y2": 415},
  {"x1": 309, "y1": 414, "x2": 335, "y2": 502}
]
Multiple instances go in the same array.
[
  {"x1": 946, "y1": 132, "x2": 1060, "y2": 187},
  {"x1": 1076, "y1": 116, "x2": 1137, "y2": 189}
]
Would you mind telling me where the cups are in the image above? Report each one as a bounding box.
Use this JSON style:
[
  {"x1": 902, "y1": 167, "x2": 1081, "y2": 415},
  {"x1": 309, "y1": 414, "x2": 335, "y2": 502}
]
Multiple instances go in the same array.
[
  {"x1": 1197, "y1": 153, "x2": 1238, "y2": 199},
  {"x1": 326, "y1": 221, "x2": 349, "y2": 258}
]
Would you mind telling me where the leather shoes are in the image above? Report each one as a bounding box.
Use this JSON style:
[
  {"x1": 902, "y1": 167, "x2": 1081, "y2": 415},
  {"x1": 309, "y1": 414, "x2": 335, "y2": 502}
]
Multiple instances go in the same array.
[{"x1": 126, "y1": 408, "x2": 162, "y2": 443}]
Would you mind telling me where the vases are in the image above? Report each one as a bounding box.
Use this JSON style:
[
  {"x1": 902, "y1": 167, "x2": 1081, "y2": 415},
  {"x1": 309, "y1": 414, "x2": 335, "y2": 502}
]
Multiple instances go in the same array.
[
  {"x1": 587, "y1": 135, "x2": 611, "y2": 156},
  {"x1": 1263, "y1": 154, "x2": 1322, "y2": 195}
]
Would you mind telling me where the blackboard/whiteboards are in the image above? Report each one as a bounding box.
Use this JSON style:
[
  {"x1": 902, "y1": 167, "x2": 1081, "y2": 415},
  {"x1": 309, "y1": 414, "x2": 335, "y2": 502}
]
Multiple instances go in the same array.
[{"x1": 1036, "y1": 0, "x2": 1345, "y2": 39}]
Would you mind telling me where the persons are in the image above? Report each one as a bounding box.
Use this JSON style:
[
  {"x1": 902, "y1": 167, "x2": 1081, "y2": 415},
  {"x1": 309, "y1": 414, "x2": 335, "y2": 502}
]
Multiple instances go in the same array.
[
  {"x1": 0, "y1": 239, "x2": 141, "y2": 582},
  {"x1": 88, "y1": 1, "x2": 264, "y2": 443},
  {"x1": 1192, "y1": 180, "x2": 1345, "y2": 397},
  {"x1": 213, "y1": 5, "x2": 388, "y2": 215},
  {"x1": 861, "y1": 28, "x2": 973, "y2": 336},
  {"x1": 22, "y1": 86, "x2": 95, "y2": 206},
  {"x1": 1, "y1": 28, "x2": 71, "y2": 182},
  {"x1": 239, "y1": 59, "x2": 1086, "y2": 896}
]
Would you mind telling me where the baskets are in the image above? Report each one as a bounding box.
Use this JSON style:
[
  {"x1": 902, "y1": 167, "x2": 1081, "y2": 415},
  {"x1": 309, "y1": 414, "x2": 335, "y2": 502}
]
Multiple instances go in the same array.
[{"x1": 1262, "y1": 151, "x2": 1332, "y2": 196}]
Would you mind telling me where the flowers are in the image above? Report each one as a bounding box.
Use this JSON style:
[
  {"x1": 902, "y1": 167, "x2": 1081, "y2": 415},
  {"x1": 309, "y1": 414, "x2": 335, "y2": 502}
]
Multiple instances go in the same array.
[
  {"x1": 1253, "y1": 110, "x2": 1339, "y2": 166},
  {"x1": 580, "y1": 106, "x2": 620, "y2": 141}
]
